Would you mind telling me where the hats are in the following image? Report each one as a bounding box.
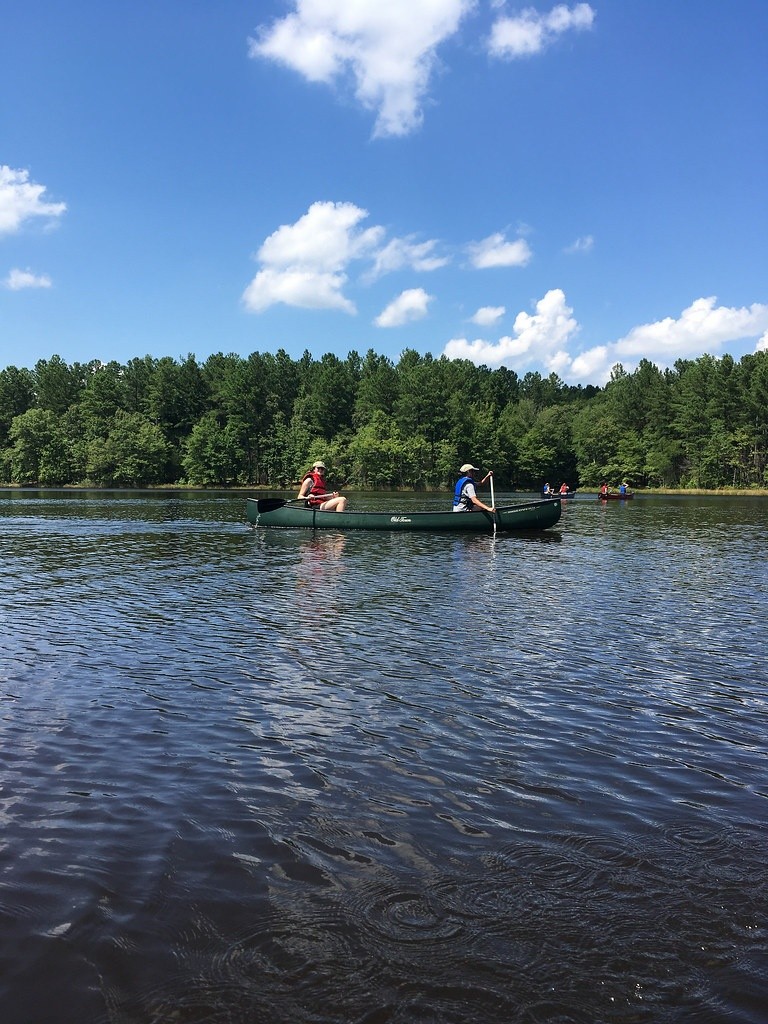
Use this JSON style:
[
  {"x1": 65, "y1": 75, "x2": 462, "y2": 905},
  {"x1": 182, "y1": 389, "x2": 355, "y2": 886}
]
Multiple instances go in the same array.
[
  {"x1": 313, "y1": 461, "x2": 326, "y2": 469},
  {"x1": 460, "y1": 464, "x2": 480, "y2": 472}
]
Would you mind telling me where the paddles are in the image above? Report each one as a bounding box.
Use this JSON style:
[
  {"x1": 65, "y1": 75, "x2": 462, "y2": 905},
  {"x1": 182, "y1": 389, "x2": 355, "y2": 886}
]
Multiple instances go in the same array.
[
  {"x1": 257, "y1": 492, "x2": 337, "y2": 514},
  {"x1": 489, "y1": 472, "x2": 497, "y2": 532}
]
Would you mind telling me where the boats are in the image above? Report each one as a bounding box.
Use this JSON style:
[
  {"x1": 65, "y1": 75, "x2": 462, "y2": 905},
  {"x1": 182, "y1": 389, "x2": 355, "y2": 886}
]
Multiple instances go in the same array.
[
  {"x1": 544, "y1": 492, "x2": 578, "y2": 500},
  {"x1": 598, "y1": 491, "x2": 630, "y2": 501},
  {"x1": 243, "y1": 495, "x2": 562, "y2": 533}
]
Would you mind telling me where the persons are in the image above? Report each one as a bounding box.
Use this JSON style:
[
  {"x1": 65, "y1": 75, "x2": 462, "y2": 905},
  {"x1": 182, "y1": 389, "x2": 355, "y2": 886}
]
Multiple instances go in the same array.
[
  {"x1": 601, "y1": 482, "x2": 608, "y2": 494},
  {"x1": 452, "y1": 464, "x2": 496, "y2": 514},
  {"x1": 544, "y1": 483, "x2": 569, "y2": 495},
  {"x1": 297, "y1": 461, "x2": 347, "y2": 512},
  {"x1": 619, "y1": 482, "x2": 629, "y2": 494}
]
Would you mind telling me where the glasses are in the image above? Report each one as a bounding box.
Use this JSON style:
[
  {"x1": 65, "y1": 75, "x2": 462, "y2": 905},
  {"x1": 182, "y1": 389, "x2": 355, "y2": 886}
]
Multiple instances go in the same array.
[{"x1": 318, "y1": 467, "x2": 325, "y2": 470}]
